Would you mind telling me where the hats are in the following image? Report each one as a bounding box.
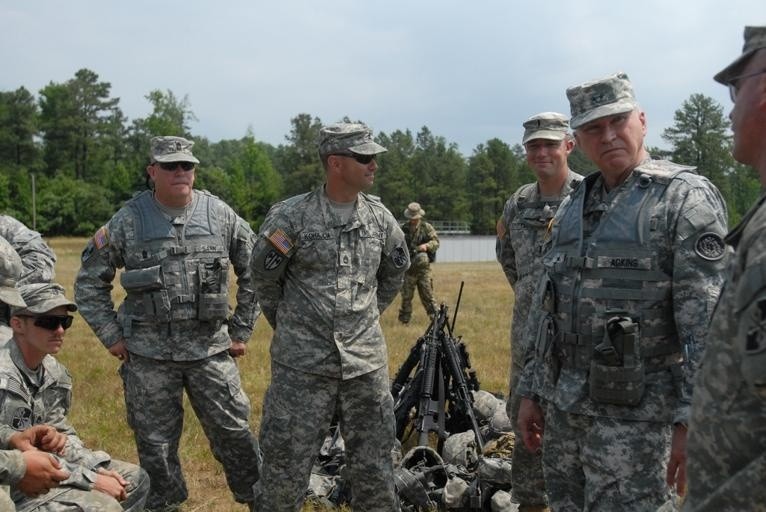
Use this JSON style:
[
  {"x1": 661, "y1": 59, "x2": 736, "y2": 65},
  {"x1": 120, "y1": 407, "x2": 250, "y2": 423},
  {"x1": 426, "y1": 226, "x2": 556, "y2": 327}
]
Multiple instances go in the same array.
[
  {"x1": 523, "y1": 72, "x2": 636, "y2": 145},
  {"x1": 319, "y1": 123, "x2": 388, "y2": 155},
  {"x1": 713, "y1": 26, "x2": 766, "y2": 85},
  {"x1": 404, "y1": 202, "x2": 425, "y2": 219},
  {"x1": 0, "y1": 235, "x2": 77, "y2": 314},
  {"x1": 150, "y1": 136, "x2": 200, "y2": 165}
]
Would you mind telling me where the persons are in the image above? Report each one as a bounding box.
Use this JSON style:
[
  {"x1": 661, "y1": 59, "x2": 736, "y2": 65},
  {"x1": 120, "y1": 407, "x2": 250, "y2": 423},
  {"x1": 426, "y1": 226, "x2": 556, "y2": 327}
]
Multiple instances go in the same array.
[
  {"x1": 250, "y1": 122, "x2": 412, "y2": 512},
  {"x1": 0, "y1": 234, "x2": 68, "y2": 510},
  {"x1": 0, "y1": 281, "x2": 149, "y2": 511},
  {"x1": 516, "y1": 74, "x2": 729, "y2": 511},
  {"x1": 74, "y1": 136, "x2": 261, "y2": 509},
  {"x1": 675, "y1": 26, "x2": 766, "y2": 512},
  {"x1": 0, "y1": 213, "x2": 56, "y2": 283},
  {"x1": 496, "y1": 111, "x2": 585, "y2": 512},
  {"x1": 398, "y1": 202, "x2": 441, "y2": 324}
]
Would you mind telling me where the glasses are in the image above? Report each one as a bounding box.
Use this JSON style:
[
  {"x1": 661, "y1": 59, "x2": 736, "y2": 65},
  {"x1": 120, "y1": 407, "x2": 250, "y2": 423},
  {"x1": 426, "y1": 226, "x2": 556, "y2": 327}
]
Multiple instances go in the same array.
[
  {"x1": 333, "y1": 153, "x2": 375, "y2": 164},
  {"x1": 16, "y1": 314, "x2": 73, "y2": 329},
  {"x1": 159, "y1": 162, "x2": 194, "y2": 170},
  {"x1": 728, "y1": 68, "x2": 766, "y2": 101}
]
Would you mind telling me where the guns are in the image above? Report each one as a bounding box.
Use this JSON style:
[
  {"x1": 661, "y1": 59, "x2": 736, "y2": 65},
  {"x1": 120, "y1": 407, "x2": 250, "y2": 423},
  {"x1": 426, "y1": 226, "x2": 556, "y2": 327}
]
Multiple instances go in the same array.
[{"x1": 390, "y1": 300, "x2": 484, "y2": 455}]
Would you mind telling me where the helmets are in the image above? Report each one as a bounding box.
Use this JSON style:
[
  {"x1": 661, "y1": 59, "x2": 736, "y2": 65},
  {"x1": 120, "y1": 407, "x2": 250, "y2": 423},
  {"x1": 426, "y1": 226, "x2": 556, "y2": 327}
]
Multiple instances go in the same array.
[{"x1": 399, "y1": 429, "x2": 478, "y2": 490}]
